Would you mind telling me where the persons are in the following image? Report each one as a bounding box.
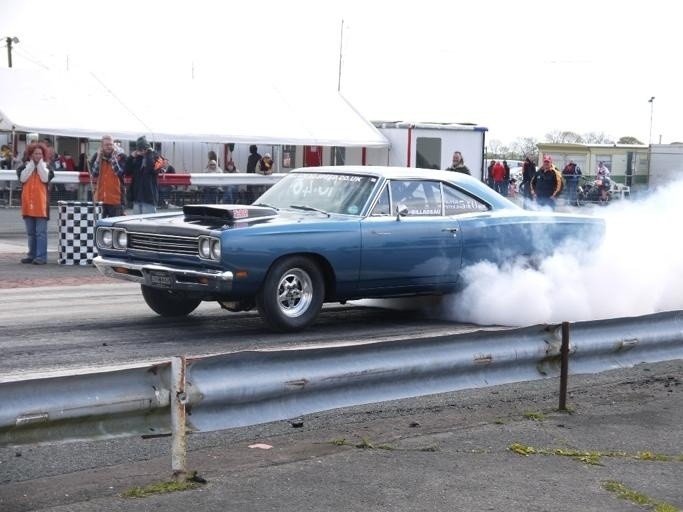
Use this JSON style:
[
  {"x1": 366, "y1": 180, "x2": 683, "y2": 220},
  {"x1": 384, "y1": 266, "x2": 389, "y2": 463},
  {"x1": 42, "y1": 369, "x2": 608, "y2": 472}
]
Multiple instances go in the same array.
[
  {"x1": 16, "y1": 142, "x2": 55, "y2": 265},
  {"x1": 254, "y1": 153, "x2": 275, "y2": 195},
  {"x1": 90, "y1": 135, "x2": 126, "y2": 218},
  {"x1": 44, "y1": 138, "x2": 50, "y2": 146},
  {"x1": 222, "y1": 160, "x2": 240, "y2": 204},
  {"x1": 0, "y1": 144, "x2": 14, "y2": 170},
  {"x1": 445, "y1": 151, "x2": 473, "y2": 175},
  {"x1": 125, "y1": 135, "x2": 165, "y2": 214},
  {"x1": 118, "y1": 152, "x2": 128, "y2": 169},
  {"x1": 203, "y1": 159, "x2": 224, "y2": 203},
  {"x1": 487, "y1": 153, "x2": 611, "y2": 212},
  {"x1": 157, "y1": 159, "x2": 175, "y2": 192}
]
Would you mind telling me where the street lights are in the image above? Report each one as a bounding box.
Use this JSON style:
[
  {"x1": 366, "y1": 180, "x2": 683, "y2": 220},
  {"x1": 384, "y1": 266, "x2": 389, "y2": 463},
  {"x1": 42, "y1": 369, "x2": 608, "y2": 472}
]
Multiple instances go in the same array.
[{"x1": 648, "y1": 96, "x2": 656, "y2": 146}]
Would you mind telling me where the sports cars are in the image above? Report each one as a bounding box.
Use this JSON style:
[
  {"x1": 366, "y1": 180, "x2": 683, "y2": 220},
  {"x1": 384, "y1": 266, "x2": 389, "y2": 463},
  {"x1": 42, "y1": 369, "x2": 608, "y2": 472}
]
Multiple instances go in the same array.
[{"x1": 91, "y1": 164, "x2": 606, "y2": 331}]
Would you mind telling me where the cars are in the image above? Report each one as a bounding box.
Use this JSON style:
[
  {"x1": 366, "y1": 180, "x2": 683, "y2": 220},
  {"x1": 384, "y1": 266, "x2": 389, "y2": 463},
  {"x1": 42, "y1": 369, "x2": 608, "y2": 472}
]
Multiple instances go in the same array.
[{"x1": 485, "y1": 159, "x2": 539, "y2": 192}]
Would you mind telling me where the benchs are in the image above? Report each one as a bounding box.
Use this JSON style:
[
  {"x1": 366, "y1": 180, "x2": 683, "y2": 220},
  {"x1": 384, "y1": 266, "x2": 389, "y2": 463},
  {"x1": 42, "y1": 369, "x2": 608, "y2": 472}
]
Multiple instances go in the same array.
[{"x1": 160, "y1": 188, "x2": 263, "y2": 205}]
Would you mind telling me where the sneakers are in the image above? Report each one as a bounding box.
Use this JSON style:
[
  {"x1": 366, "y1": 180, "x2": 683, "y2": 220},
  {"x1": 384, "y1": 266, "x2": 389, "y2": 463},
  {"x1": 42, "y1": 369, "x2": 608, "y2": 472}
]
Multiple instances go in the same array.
[
  {"x1": 32, "y1": 259, "x2": 46, "y2": 264},
  {"x1": 21, "y1": 259, "x2": 32, "y2": 263}
]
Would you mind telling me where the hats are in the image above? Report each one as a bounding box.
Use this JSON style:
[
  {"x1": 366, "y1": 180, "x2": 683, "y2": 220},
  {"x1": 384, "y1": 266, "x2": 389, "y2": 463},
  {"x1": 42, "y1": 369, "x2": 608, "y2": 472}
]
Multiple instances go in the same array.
[
  {"x1": 136, "y1": 136, "x2": 151, "y2": 148},
  {"x1": 543, "y1": 156, "x2": 551, "y2": 162}
]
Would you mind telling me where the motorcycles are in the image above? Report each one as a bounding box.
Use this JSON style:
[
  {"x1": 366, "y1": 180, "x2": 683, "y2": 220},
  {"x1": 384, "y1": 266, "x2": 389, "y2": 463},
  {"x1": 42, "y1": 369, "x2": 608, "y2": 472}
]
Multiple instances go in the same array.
[{"x1": 574, "y1": 178, "x2": 611, "y2": 206}]
